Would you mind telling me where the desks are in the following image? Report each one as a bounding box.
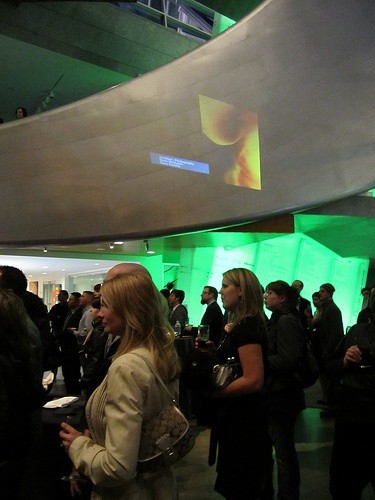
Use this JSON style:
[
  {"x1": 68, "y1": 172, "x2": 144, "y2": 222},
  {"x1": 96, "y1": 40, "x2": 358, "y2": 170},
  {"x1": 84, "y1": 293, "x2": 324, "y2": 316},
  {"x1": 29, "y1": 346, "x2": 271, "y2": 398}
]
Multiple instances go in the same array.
[{"x1": 175, "y1": 334, "x2": 197, "y2": 419}]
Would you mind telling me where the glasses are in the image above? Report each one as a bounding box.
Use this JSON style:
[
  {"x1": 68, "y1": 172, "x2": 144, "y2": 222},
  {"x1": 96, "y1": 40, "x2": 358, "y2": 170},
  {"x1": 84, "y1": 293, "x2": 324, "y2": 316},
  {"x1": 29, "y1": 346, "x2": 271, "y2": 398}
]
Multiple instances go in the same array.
[{"x1": 202, "y1": 292, "x2": 209, "y2": 294}]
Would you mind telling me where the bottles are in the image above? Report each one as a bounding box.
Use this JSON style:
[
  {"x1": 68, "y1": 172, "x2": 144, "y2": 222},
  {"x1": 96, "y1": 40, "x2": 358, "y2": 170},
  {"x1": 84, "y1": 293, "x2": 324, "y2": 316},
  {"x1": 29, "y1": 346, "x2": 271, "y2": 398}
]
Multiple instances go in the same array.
[{"x1": 174, "y1": 321, "x2": 181, "y2": 336}]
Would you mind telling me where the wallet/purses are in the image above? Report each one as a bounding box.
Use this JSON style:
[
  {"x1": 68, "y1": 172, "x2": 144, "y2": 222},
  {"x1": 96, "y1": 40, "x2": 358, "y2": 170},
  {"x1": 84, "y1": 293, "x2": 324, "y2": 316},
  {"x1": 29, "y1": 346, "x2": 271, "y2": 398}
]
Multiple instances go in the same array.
[{"x1": 348, "y1": 348, "x2": 375, "y2": 373}]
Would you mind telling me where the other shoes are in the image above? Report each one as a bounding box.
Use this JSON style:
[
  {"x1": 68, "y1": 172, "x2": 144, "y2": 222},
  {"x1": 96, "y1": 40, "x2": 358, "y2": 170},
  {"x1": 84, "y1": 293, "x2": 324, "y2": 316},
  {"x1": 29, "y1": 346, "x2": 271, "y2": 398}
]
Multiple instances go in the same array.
[
  {"x1": 320, "y1": 410, "x2": 335, "y2": 419},
  {"x1": 317, "y1": 399, "x2": 327, "y2": 405}
]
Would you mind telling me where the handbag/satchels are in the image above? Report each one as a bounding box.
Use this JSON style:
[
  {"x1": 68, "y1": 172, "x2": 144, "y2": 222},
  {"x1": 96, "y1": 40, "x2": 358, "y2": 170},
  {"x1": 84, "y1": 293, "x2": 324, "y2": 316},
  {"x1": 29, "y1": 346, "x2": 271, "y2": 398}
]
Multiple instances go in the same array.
[
  {"x1": 272, "y1": 315, "x2": 318, "y2": 391},
  {"x1": 212, "y1": 357, "x2": 243, "y2": 388},
  {"x1": 98, "y1": 353, "x2": 195, "y2": 476}
]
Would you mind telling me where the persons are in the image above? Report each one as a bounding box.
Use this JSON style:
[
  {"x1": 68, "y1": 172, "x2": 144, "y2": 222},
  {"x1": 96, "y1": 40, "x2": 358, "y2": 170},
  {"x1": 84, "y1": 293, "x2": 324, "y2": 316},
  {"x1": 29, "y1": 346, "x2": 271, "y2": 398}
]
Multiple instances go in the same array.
[{"x1": 0, "y1": 263, "x2": 375, "y2": 500}]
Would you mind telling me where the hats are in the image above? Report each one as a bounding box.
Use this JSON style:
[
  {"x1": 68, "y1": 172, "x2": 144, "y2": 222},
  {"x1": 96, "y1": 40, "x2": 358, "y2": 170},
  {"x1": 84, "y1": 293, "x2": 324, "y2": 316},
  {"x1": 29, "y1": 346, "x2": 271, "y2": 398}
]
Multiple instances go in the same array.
[
  {"x1": 361, "y1": 287, "x2": 371, "y2": 294},
  {"x1": 320, "y1": 283, "x2": 335, "y2": 296}
]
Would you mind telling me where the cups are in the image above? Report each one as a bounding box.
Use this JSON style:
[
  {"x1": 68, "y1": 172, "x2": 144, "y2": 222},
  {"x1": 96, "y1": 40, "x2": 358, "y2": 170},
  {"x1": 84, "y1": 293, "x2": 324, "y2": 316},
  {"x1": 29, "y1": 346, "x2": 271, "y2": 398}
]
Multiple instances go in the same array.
[
  {"x1": 60, "y1": 403, "x2": 82, "y2": 447},
  {"x1": 354, "y1": 335, "x2": 371, "y2": 368},
  {"x1": 198, "y1": 325, "x2": 209, "y2": 352}
]
